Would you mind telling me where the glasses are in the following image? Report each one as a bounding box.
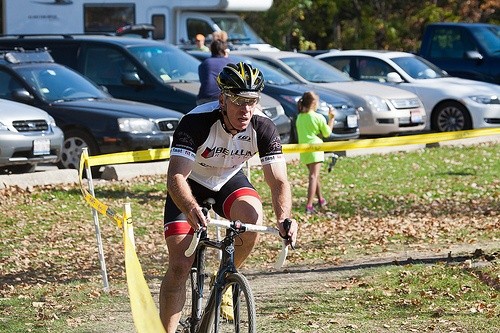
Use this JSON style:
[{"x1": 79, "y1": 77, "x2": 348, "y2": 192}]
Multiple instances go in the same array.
[{"x1": 224, "y1": 92, "x2": 258, "y2": 106}]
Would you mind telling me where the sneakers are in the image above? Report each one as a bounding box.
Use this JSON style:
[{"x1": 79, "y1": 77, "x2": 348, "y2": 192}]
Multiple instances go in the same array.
[{"x1": 209, "y1": 272, "x2": 234, "y2": 324}]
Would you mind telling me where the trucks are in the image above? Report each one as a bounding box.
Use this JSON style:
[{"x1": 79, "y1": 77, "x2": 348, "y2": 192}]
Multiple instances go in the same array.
[{"x1": 0, "y1": 0, "x2": 280, "y2": 51}]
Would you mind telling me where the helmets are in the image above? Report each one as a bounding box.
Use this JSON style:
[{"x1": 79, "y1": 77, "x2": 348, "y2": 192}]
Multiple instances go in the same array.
[{"x1": 217, "y1": 62, "x2": 264, "y2": 98}]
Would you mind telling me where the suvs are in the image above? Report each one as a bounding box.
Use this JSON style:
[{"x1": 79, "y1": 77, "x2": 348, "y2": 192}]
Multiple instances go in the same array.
[{"x1": 0, "y1": 32, "x2": 292, "y2": 145}]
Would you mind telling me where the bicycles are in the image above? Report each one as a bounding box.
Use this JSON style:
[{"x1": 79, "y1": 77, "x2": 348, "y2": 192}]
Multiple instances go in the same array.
[{"x1": 176, "y1": 198, "x2": 292, "y2": 333}]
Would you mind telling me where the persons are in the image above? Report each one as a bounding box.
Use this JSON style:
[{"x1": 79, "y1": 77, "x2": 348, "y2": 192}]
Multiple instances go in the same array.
[
  {"x1": 159, "y1": 63, "x2": 299, "y2": 333},
  {"x1": 194, "y1": 31, "x2": 234, "y2": 105},
  {"x1": 296, "y1": 92, "x2": 337, "y2": 214}
]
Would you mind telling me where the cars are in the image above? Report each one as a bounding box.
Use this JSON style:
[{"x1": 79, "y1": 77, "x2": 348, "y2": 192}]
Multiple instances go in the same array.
[
  {"x1": 313, "y1": 50, "x2": 500, "y2": 133},
  {"x1": 230, "y1": 50, "x2": 427, "y2": 139},
  {"x1": 0, "y1": 47, "x2": 186, "y2": 179},
  {"x1": 183, "y1": 49, "x2": 361, "y2": 142},
  {"x1": 0, "y1": 98, "x2": 65, "y2": 175}
]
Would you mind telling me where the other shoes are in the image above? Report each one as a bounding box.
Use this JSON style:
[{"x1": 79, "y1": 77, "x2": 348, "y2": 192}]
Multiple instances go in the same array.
[
  {"x1": 318, "y1": 199, "x2": 326, "y2": 207},
  {"x1": 305, "y1": 205, "x2": 313, "y2": 213}
]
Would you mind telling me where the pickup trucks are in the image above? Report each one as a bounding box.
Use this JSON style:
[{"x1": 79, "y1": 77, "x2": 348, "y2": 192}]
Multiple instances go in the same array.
[{"x1": 413, "y1": 22, "x2": 500, "y2": 85}]
